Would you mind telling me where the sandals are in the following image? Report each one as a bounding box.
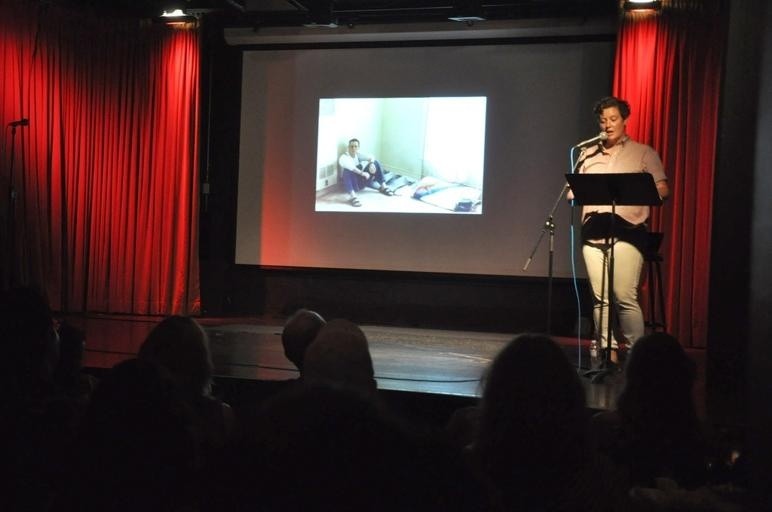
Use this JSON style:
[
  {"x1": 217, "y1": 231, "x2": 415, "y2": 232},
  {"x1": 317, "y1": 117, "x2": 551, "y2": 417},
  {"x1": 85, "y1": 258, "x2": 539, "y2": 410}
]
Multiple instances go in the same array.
[
  {"x1": 350, "y1": 196, "x2": 360, "y2": 206},
  {"x1": 380, "y1": 188, "x2": 393, "y2": 196}
]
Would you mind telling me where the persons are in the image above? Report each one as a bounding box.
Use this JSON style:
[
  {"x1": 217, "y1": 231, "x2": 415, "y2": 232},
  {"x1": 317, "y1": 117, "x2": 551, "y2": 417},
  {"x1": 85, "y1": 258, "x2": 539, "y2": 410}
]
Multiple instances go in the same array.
[
  {"x1": 566, "y1": 97, "x2": 670, "y2": 365},
  {"x1": 340, "y1": 139, "x2": 392, "y2": 208},
  {"x1": 446, "y1": 334, "x2": 629, "y2": 511},
  {"x1": 1, "y1": 272, "x2": 109, "y2": 511},
  {"x1": 243, "y1": 310, "x2": 436, "y2": 511},
  {"x1": 105, "y1": 314, "x2": 237, "y2": 512},
  {"x1": 593, "y1": 333, "x2": 706, "y2": 491}
]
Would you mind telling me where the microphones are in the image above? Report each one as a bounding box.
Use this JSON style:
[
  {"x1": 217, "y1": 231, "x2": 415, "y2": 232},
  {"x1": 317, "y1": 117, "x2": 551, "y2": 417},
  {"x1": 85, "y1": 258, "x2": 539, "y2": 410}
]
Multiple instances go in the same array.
[
  {"x1": 574, "y1": 131, "x2": 608, "y2": 151},
  {"x1": 7, "y1": 118, "x2": 29, "y2": 127}
]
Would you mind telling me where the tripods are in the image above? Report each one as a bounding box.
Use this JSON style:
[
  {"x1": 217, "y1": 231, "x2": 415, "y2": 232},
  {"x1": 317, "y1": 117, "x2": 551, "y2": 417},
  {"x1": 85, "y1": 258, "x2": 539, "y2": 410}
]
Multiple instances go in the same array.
[{"x1": 583, "y1": 201, "x2": 624, "y2": 384}]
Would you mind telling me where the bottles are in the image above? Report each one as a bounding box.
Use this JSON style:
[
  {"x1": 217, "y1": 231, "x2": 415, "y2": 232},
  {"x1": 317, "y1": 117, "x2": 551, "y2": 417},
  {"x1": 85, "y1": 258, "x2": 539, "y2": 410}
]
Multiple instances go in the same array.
[{"x1": 589, "y1": 339, "x2": 600, "y2": 368}]
